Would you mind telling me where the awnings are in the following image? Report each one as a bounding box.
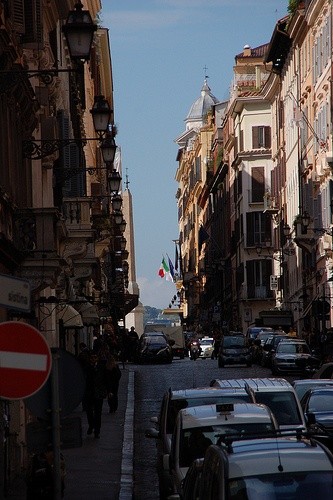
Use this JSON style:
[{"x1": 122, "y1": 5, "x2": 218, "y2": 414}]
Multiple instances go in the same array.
[{"x1": 56, "y1": 297, "x2": 100, "y2": 329}]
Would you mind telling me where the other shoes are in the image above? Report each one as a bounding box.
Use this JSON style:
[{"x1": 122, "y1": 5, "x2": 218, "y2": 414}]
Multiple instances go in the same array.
[
  {"x1": 95, "y1": 432, "x2": 100, "y2": 438},
  {"x1": 87, "y1": 424, "x2": 92, "y2": 435}
]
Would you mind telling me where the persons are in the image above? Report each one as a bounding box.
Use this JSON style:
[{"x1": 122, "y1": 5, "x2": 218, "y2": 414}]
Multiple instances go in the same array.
[
  {"x1": 185, "y1": 327, "x2": 307, "y2": 361},
  {"x1": 28, "y1": 325, "x2": 138, "y2": 500}
]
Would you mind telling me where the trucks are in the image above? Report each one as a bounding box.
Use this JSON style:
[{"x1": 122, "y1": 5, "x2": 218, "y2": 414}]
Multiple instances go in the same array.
[{"x1": 153, "y1": 327, "x2": 185, "y2": 359}]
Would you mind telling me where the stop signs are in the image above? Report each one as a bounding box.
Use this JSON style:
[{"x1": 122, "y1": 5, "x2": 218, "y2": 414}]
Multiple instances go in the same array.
[{"x1": 0, "y1": 321, "x2": 51, "y2": 399}]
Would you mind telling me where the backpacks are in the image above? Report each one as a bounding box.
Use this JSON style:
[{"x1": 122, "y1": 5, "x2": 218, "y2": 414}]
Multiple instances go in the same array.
[{"x1": 30, "y1": 454, "x2": 55, "y2": 488}]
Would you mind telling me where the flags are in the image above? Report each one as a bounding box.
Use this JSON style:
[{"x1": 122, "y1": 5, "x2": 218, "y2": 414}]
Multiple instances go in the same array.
[{"x1": 157, "y1": 256, "x2": 179, "y2": 282}]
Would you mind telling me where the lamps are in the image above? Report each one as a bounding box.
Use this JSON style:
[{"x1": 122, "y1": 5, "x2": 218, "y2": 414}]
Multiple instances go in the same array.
[
  {"x1": 169, "y1": 261, "x2": 228, "y2": 308},
  {"x1": 302, "y1": 211, "x2": 310, "y2": 234},
  {"x1": 283, "y1": 221, "x2": 295, "y2": 239},
  {"x1": 0, "y1": 0, "x2": 130, "y2": 288},
  {"x1": 255, "y1": 246, "x2": 273, "y2": 257},
  {"x1": 265, "y1": 238, "x2": 281, "y2": 252}
]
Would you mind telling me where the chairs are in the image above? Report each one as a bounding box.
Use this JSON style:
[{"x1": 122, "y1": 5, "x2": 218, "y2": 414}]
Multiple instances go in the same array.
[{"x1": 180, "y1": 430, "x2": 214, "y2": 469}]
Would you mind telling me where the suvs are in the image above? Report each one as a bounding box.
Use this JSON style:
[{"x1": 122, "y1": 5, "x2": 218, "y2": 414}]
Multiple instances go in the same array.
[{"x1": 146, "y1": 384, "x2": 256, "y2": 473}]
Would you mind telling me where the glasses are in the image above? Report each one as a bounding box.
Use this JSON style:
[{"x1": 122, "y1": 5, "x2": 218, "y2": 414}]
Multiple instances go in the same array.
[{"x1": 90, "y1": 356, "x2": 96, "y2": 359}]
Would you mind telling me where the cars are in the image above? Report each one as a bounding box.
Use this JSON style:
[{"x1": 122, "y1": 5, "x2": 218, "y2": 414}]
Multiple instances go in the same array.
[
  {"x1": 292, "y1": 378, "x2": 332, "y2": 404},
  {"x1": 167, "y1": 458, "x2": 204, "y2": 500},
  {"x1": 218, "y1": 335, "x2": 253, "y2": 368},
  {"x1": 189, "y1": 337, "x2": 215, "y2": 360},
  {"x1": 300, "y1": 387, "x2": 333, "y2": 436},
  {"x1": 246, "y1": 327, "x2": 305, "y2": 367},
  {"x1": 201, "y1": 428, "x2": 333, "y2": 500},
  {"x1": 162, "y1": 403, "x2": 281, "y2": 495},
  {"x1": 271, "y1": 341, "x2": 316, "y2": 378},
  {"x1": 210, "y1": 377, "x2": 315, "y2": 437},
  {"x1": 139, "y1": 332, "x2": 174, "y2": 365}
]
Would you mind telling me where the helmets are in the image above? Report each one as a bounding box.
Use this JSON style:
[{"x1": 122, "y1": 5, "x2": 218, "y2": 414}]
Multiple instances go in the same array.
[
  {"x1": 253, "y1": 338, "x2": 261, "y2": 344},
  {"x1": 248, "y1": 338, "x2": 254, "y2": 344}
]
